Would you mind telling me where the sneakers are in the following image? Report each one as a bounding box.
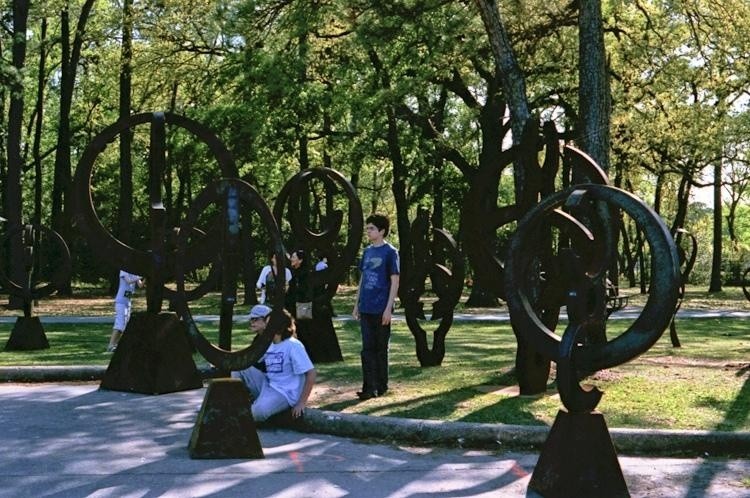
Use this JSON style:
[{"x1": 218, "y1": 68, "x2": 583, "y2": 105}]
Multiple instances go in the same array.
[
  {"x1": 356, "y1": 384, "x2": 389, "y2": 400},
  {"x1": 107, "y1": 343, "x2": 118, "y2": 351}
]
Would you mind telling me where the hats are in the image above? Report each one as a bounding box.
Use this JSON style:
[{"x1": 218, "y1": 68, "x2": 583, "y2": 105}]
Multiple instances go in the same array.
[{"x1": 245, "y1": 305, "x2": 273, "y2": 321}]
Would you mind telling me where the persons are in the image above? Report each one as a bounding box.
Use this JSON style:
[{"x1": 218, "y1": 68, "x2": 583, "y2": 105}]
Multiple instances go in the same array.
[
  {"x1": 246, "y1": 304, "x2": 274, "y2": 373},
  {"x1": 350, "y1": 213, "x2": 402, "y2": 401},
  {"x1": 315, "y1": 257, "x2": 329, "y2": 271},
  {"x1": 105, "y1": 269, "x2": 147, "y2": 352},
  {"x1": 256, "y1": 249, "x2": 292, "y2": 302},
  {"x1": 229, "y1": 308, "x2": 317, "y2": 425},
  {"x1": 284, "y1": 248, "x2": 313, "y2": 326}
]
